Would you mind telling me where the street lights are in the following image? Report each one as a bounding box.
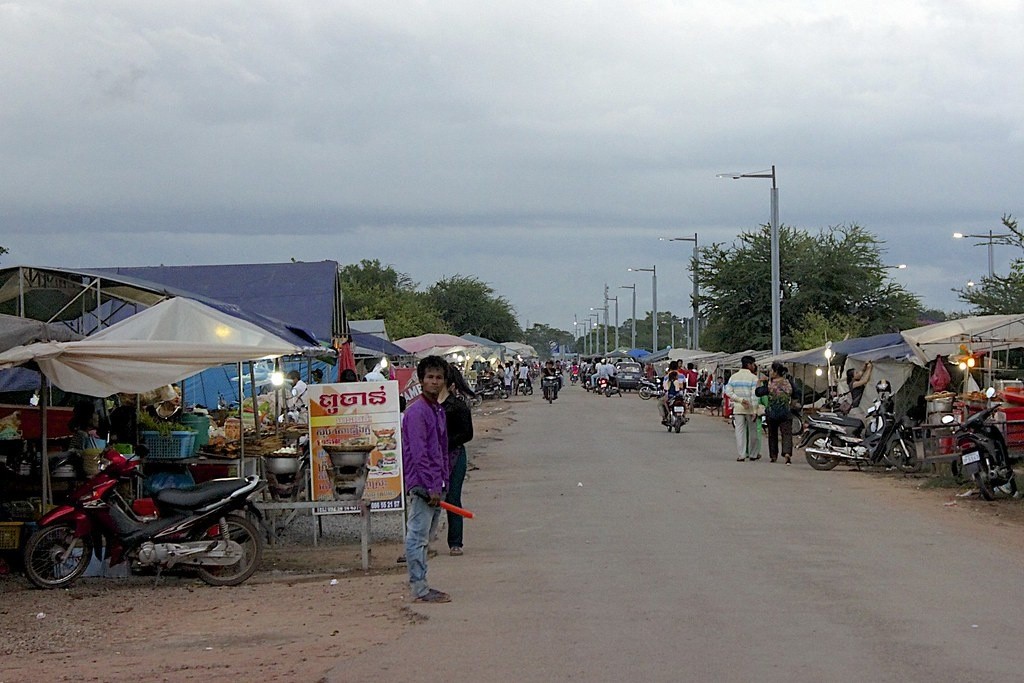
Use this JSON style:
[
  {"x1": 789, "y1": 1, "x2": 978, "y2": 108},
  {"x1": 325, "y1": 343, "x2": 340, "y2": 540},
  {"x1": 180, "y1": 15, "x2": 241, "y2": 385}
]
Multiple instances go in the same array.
[
  {"x1": 715, "y1": 166, "x2": 782, "y2": 356},
  {"x1": 863, "y1": 264, "x2": 906, "y2": 294},
  {"x1": 953, "y1": 231, "x2": 1016, "y2": 308},
  {"x1": 573, "y1": 233, "x2": 698, "y2": 356}
]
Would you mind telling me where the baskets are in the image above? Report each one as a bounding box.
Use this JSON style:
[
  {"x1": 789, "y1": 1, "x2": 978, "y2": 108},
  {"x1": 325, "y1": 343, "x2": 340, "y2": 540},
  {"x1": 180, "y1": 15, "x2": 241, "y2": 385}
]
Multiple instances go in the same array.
[
  {"x1": 189, "y1": 431, "x2": 199, "y2": 458},
  {"x1": 138, "y1": 429, "x2": 191, "y2": 460}
]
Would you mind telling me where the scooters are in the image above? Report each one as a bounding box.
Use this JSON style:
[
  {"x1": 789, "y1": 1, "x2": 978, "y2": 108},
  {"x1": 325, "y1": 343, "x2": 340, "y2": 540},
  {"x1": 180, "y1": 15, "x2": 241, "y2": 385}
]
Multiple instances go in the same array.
[
  {"x1": 600, "y1": 365, "x2": 622, "y2": 397},
  {"x1": 569, "y1": 370, "x2": 578, "y2": 384}
]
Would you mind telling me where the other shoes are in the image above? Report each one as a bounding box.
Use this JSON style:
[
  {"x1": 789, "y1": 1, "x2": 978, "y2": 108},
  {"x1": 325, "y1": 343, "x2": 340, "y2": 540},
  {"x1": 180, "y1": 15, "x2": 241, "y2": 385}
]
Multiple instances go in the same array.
[
  {"x1": 784, "y1": 454, "x2": 792, "y2": 465},
  {"x1": 771, "y1": 457, "x2": 777, "y2": 462},
  {"x1": 750, "y1": 455, "x2": 762, "y2": 460},
  {"x1": 450, "y1": 546, "x2": 464, "y2": 557},
  {"x1": 737, "y1": 458, "x2": 746, "y2": 461},
  {"x1": 415, "y1": 589, "x2": 451, "y2": 603},
  {"x1": 662, "y1": 416, "x2": 668, "y2": 422}
]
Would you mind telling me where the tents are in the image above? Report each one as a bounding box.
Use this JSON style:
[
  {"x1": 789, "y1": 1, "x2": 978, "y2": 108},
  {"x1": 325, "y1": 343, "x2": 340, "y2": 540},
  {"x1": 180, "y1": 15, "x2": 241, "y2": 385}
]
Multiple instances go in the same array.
[
  {"x1": 347, "y1": 320, "x2": 537, "y2": 360},
  {"x1": 724, "y1": 314, "x2": 1024, "y2": 367},
  {"x1": 0, "y1": 265, "x2": 336, "y2": 521}
]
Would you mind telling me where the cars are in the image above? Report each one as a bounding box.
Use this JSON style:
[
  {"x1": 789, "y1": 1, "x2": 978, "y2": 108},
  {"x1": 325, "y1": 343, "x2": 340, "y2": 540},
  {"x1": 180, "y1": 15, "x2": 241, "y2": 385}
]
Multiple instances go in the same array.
[{"x1": 616, "y1": 361, "x2": 644, "y2": 390}]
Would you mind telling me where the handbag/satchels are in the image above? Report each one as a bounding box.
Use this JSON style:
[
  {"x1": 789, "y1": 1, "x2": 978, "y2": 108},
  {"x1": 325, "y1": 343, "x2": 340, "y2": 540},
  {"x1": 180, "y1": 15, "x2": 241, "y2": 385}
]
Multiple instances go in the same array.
[
  {"x1": 969, "y1": 373, "x2": 981, "y2": 395},
  {"x1": 929, "y1": 354, "x2": 950, "y2": 392}
]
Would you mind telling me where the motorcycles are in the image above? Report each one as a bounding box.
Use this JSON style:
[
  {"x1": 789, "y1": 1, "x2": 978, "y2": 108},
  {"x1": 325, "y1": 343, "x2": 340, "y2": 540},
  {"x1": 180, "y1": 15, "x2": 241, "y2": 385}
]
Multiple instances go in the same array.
[
  {"x1": 942, "y1": 387, "x2": 1018, "y2": 502},
  {"x1": 469, "y1": 373, "x2": 534, "y2": 407},
  {"x1": 24, "y1": 432, "x2": 261, "y2": 592},
  {"x1": 661, "y1": 397, "x2": 689, "y2": 432},
  {"x1": 794, "y1": 379, "x2": 921, "y2": 473},
  {"x1": 541, "y1": 372, "x2": 562, "y2": 403},
  {"x1": 728, "y1": 406, "x2": 803, "y2": 437},
  {"x1": 638, "y1": 377, "x2": 662, "y2": 400}
]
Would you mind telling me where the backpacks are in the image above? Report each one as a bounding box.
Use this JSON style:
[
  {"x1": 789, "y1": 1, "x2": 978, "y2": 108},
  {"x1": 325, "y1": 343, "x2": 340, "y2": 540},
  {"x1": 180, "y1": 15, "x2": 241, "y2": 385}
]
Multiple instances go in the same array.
[{"x1": 840, "y1": 392, "x2": 854, "y2": 415}]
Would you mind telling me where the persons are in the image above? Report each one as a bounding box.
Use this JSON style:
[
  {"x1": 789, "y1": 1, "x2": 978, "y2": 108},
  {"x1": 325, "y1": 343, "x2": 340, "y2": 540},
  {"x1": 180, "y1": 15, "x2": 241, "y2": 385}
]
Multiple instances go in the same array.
[
  {"x1": 755, "y1": 363, "x2": 797, "y2": 464},
  {"x1": 746, "y1": 364, "x2": 768, "y2": 460},
  {"x1": 846, "y1": 358, "x2": 872, "y2": 417},
  {"x1": 403, "y1": 355, "x2": 470, "y2": 604},
  {"x1": 339, "y1": 369, "x2": 357, "y2": 383},
  {"x1": 647, "y1": 360, "x2": 723, "y2": 426},
  {"x1": 472, "y1": 357, "x2": 625, "y2": 400},
  {"x1": 428, "y1": 361, "x2": 477, "y2": 556},
  {"x1": 725, "y1": 355, "x2": 760, "y2": 461}
]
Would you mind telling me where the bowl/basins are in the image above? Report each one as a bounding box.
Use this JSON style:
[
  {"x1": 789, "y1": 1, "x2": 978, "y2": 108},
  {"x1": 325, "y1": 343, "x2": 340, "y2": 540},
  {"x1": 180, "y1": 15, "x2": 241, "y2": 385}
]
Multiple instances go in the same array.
[{"x1": 327, "y1": 450, "x2": 369, "y2": 468}]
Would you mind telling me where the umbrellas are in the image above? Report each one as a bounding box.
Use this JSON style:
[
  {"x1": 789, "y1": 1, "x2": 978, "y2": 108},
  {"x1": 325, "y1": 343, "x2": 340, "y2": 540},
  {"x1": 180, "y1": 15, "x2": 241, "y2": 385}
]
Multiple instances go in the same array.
[{"x1": 605, "y1": 346, "x2": 723, "y2": 364}]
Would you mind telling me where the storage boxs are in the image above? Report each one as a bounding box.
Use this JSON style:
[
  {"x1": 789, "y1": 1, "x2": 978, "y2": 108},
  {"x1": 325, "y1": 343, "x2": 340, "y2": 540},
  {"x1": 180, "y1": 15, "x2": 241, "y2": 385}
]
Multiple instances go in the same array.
[
  {"x1": 142, "y1": 428, "x2": 198, "y2": 460},
  {"x1": 0, "y1": 521, "x2": 24, "y2": 549}
]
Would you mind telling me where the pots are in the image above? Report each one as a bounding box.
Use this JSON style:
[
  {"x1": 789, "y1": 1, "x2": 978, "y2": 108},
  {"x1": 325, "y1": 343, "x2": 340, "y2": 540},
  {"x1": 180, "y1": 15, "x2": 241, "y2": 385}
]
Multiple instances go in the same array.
[
  {"x1": 50, "y1": 463, "x2": 80, "y2": 479},
  {"x1": 261, "y1": 451, "x2": 306, "y2": 475},
  {"x1": 7, "y1": 458, "x2": 33, "y2": 477},
  {"x1": 927, "y1": 395, "x2": 955, "y2": 414}
]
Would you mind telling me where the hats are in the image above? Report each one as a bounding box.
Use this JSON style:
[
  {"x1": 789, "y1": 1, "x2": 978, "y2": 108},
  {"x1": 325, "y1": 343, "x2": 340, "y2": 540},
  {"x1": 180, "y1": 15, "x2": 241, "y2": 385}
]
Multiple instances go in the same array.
[{"x1": 311, "y1": 368, "x2": 323, "y2": 379}]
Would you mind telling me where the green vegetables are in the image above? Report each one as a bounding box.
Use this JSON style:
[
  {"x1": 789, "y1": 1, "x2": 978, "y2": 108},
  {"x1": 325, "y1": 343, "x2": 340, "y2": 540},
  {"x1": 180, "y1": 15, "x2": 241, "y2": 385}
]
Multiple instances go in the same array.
[{"x1": 136, "y1": 407, "x2": 196, "y2": 452}]
